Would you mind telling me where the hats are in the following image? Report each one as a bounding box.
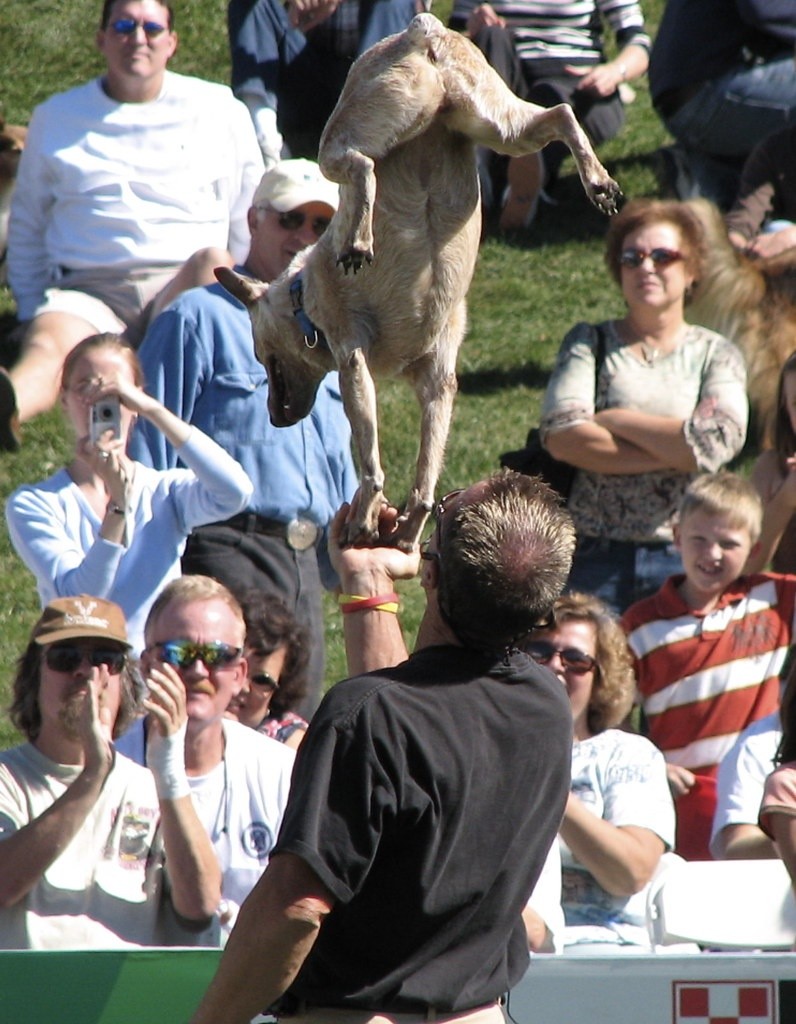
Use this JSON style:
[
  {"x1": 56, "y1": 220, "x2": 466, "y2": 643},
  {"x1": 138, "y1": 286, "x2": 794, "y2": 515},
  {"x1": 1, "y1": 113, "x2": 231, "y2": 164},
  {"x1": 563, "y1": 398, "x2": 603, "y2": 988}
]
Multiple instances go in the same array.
[
  {"x1": 28, "y1": 592, "x2": 135, "y2": 651},
  {"x1": 252, "y1": 156, "x2": 339, "y2": 217}
]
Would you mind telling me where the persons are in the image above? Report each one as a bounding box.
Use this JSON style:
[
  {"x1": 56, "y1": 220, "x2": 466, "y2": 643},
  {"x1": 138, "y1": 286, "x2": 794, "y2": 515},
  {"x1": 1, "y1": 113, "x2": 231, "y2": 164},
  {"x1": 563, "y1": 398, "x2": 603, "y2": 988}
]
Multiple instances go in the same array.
[
  {"x1": 620, "y1": 472, "x2": 796, "y2": 861},
  {"x1": 710, "y1": 645, "x2": 796, "y2": 883},
  {"x1": 193, "y1": 469, "x2": 575, "y2": 1024},
  {"x1": 1, "y1": 332, "x2": 310, "y2": 951},
  {"x1": 520, "y1": 591, "x2": 672, "y2": 958},
  {"x1": 126, "y1": 159, "x2": 356, "y2": 717},
  {"x1": 227, "y1": 0, "x2": 434, "y2": 162},
  {"x1": 0, "y1": 0, "x2": 265, "y2": 452},
  {"x1": 448, "y1": 0, "x2": 652, "y2": 234},
  {"x1": 540, "y1": 200, "x2": 748, "y2": 617},
  {"x1": 746, "y1": 348, "x2": 796, "y2": 576},
  {"x1": 650, "y1": 0, "x2": 796, "y2": 257}
]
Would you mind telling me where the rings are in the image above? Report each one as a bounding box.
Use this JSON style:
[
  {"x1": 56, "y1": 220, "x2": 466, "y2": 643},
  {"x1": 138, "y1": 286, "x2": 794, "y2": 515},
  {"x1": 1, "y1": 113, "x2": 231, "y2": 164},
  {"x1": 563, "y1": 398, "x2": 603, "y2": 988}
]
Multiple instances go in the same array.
[{"x1": 98, "y1": 452, "x2": 108, "y2": 456}]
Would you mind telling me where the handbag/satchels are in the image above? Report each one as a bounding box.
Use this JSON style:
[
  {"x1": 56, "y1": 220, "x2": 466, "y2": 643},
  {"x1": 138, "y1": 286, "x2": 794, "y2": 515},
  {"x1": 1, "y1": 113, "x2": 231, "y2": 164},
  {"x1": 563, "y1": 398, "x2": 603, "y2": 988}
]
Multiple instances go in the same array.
[{"x1": 499, "y1": 321, "x2": 605, "y2": 501}]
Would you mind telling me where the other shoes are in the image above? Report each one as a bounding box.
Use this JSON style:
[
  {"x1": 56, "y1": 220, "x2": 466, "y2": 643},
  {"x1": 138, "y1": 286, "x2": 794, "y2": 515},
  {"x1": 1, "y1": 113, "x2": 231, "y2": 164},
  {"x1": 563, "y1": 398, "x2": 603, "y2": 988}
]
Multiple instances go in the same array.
[
  {"x1": 497, "y1": 149, "x2": 546, "y2": 234},
  {"x1": 0, "y1": 369, "x2": 24, "y2": 452}
]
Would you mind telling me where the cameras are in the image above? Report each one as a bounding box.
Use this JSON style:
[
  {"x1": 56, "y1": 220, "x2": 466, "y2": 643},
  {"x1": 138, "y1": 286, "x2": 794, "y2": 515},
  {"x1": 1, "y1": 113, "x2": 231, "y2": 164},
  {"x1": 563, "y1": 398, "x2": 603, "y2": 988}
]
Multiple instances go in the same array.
[{"x1": 88, "y1": 394, "x2": 120, "y2": 448}]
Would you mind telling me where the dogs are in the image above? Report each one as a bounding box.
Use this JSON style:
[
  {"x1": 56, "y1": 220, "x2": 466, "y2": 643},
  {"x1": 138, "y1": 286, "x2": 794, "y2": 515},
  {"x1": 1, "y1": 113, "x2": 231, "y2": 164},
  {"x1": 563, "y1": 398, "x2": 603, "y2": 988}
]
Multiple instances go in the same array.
[
  {"x1": 682, "y1": 198, "x2": 795, "y2": 453},
  {"x1": 214, "y1": 13, "x2": 624, "y2": 553}
]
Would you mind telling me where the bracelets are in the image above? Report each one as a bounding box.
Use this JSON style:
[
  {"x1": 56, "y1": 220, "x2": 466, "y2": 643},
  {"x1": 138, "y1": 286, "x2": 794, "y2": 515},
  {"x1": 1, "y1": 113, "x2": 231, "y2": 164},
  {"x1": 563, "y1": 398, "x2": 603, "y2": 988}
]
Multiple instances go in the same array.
[
  {"x1": 106, "y1": 502, "x2": 126, "y2": 515},
  {"x1": 338, "y1": 592, "x2": 398, "y2": 614}
]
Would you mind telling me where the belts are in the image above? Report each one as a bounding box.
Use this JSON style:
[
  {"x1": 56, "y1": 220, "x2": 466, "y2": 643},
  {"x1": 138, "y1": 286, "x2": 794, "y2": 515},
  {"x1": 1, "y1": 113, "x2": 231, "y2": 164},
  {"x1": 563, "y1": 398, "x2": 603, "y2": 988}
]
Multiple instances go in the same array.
[{"x1": 228, "y1": 513, "x2": 324, "y2": 550}]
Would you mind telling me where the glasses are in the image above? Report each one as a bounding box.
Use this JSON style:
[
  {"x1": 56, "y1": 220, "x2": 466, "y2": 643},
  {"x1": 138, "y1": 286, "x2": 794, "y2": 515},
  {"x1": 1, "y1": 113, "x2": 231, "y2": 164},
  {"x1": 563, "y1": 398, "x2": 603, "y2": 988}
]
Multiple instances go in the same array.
[
  {"x1": 248, "y1": 671, "x2": 282, "y2": 693},
  {"x1": 146, "y1": 638, "x2": 243, "y2": 669},
  {"x1": 431, "y1": 488, "x2": 463, "y2": 550},
  {"x1": 522, "y1": 642, "x2": 597, "y2": 675},
  {"x1": 109, "y1": 17, "x2": 165, "y2": 38},
  {"x1": 258, "y1": 207, "x2": 330, "y2": 236},
  {"x1": 35, "y1": 646, "x2": 129, "y2": 676},
  {"x1": 619, "y1": 247, "x2": 689, "y2": 267}
]
